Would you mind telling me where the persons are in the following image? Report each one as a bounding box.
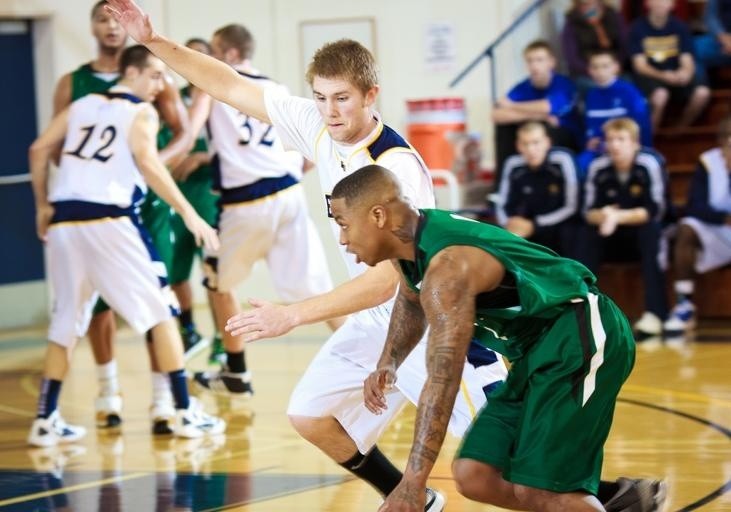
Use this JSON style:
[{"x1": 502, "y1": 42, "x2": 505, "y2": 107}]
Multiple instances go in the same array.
[
  {"x1": 328, "y1": 161, "x2": 667, "y2": 512},
  {"x1": 28, "y1": 46, "x2": 228, "y2": 445},
  {"x1": 28, "y1": 433, "x2": 232, "y2": 511},
  {"x1": 488, "y1": 1, "x2": 731, "y2": 335},
  {"x1": 98, "y1": 4, "x2": 509, "y2": 512},
  {"x1": 31, "y1": 386, "x2": 252, "y2": 511},
  {"x1": 174, "y1": 40, "x2": 228, "y2": 378},
  {"x1": 158, "y1": 26, "x2": 350, "y2": 398},
  {"x1": 50, "y1": 2, "x2": 195, "y2": 426}
]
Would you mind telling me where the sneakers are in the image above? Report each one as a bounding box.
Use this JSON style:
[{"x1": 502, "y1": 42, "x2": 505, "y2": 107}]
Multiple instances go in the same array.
[
  {"x1": 660, "y1": 301, "x2": 699, "y2": 334},
  {"x1": 201, "y1": 352, "x2": 228, "y2": 380},
  {"x1": 168, "y1": 396, "x2": 226, "y2": 439},
  {"x1": 147, "y1": 406, "x2": 173, "y2": 434},
  {"x1": 193, "y1": 370, "x2": 254, "y2": 394},
  {"x1": 603, "y1": 476, "x2": 671, "y2": 512},
  {"x1": 181, "y1": 328, "x2": 208, "y2": 362},
  {"x1": 632, "y1": 310, "x2": 662, "y2": 337},
  {"x1": 28, "y1": 409, "x2": 86, "y2": 447},
  {"x1": 425, "y1": 487, "x2": 446, "y2": 512},
  {"x1": 94, "y1": 395, "x2": 122, "y2": 431}
]
